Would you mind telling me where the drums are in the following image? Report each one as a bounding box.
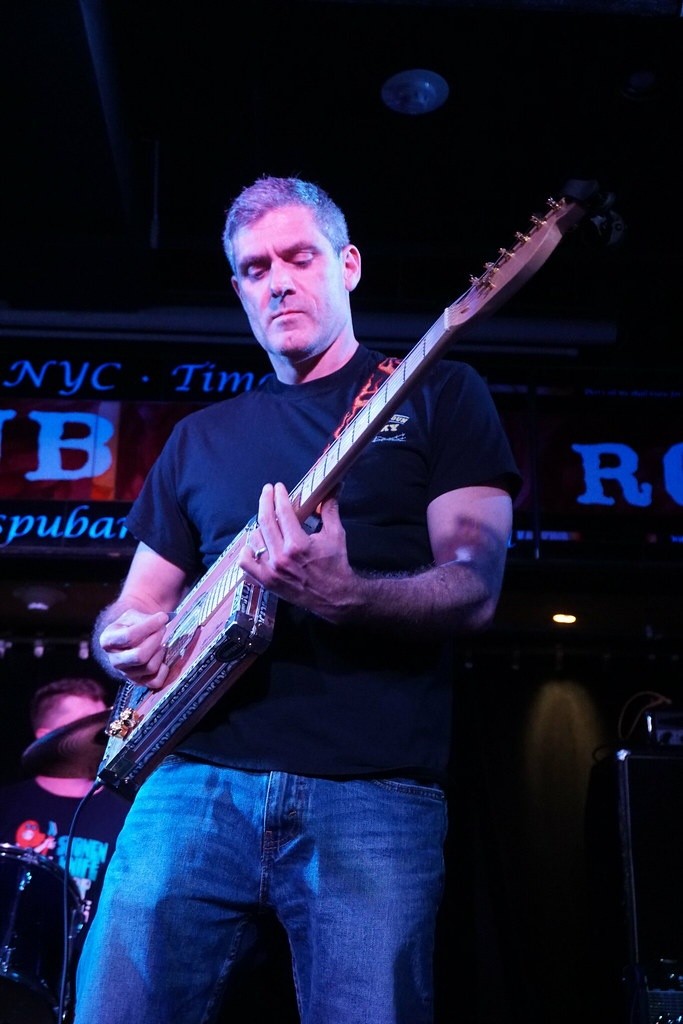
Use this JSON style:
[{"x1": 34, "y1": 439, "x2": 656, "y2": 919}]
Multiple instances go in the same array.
[{"x1": 0, "y1": 843, "x2": 82, "y2": 1024}]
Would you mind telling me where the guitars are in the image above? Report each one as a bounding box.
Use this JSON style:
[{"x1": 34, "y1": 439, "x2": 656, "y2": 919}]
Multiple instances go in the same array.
[{"x1": 95, "y1": 193, "x2": 580, "y2": 798}]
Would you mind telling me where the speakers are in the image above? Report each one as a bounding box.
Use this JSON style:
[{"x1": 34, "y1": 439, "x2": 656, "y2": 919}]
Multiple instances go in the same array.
[{"x1": 580, "y1": 749, "x2": 682, "y2": 1024}]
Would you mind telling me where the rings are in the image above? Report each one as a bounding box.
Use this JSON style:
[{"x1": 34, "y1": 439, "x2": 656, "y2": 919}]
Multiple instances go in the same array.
[{"x1": 255, "y1": 547, "x2": 267, "y2": 562}]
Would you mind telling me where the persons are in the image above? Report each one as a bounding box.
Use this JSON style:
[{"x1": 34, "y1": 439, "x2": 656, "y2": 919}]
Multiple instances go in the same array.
[
  {"x1": 0, "y1": 679, "x2": 132, "y2": 937},
  {"x1": 73, "y1": 177, "x2": 523, "y2": 1024}
]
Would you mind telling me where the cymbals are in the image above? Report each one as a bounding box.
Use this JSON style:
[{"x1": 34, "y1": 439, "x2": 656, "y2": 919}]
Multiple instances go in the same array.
[{"x1": 23, "y1": 706, "x2": 113, "y2": 779}]
